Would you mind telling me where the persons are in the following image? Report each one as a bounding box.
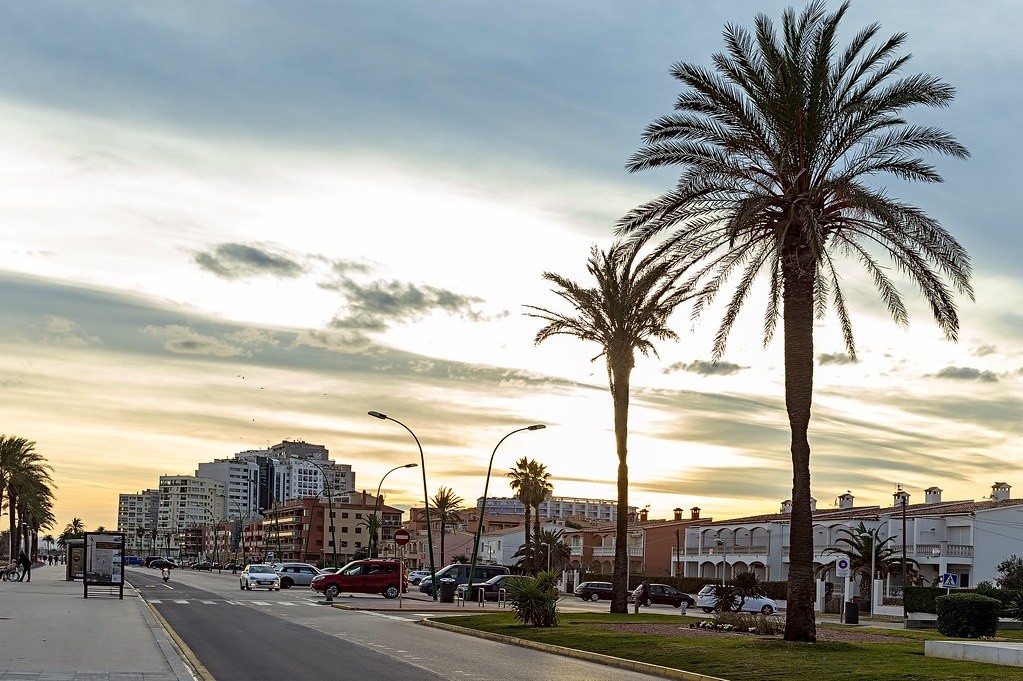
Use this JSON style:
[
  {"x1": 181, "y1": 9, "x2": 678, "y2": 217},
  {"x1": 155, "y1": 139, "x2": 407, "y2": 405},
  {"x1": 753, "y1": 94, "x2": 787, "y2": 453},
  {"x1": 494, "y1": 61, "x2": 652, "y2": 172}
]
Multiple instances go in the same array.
[
  {"x1": 54, "y1": 556, "x2": 58, "y2": 565},
  {"x1": 0, "y1": 559, "x2": 16, "y2": 581},
  {"x1": 43, "y1": 555, "x2": 47, "y2": 562},
  {"x1": 48, "y1": 555, "x2": 52, "y2": 565},
  {"x1": 160, "y1": 560, "x2": 171, "y2": 575},
  {"x1": 641, "y1": 578, "x2": 650, "y2": 606},
  {"x1": 17, "y1": 551, "x2": 31, "y2": 582},
  {"x1": 39, "y1": 555, "x2": 42, "y2": 560}
]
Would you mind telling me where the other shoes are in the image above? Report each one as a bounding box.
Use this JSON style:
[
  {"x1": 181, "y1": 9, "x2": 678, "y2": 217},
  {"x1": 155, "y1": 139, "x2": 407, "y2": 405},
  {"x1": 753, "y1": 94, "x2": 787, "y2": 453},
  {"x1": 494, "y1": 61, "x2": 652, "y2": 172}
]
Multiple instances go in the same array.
[
  {"x1": 26, "y1": 580, "x2": 30, "y2": 582},
  {"x1": 18, "y1": 580, "x2": 22, "y2": 582}
]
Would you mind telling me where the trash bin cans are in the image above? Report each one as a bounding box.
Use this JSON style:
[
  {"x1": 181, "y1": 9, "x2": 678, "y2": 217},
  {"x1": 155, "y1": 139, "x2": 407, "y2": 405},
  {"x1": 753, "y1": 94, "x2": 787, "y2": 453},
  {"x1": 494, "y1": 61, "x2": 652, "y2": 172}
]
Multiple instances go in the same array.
[
  {"x1": 440, "y1": 578, "x2": 455, "y2": 603},
  {"x1": 845, "y1": 602, "x2": 858, "y2": 624}
]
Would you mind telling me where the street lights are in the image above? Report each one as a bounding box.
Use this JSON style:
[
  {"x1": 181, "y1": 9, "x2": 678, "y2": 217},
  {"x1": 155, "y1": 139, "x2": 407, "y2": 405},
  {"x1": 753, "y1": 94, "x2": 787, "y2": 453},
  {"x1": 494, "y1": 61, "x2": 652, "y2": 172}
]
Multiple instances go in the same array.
[
  {"x1": 289, "y1": 454, "x2": 338, "y2": 573},
  {"x1": 859, "y1": 529, "x2": 875, "y2": 618},
  {"x1": 184, "y1": 512, "x2": 201, "y2": 572},
  {"x1": 304, "y1": 485, "x2": 334, "y2": 564},
  {"x1": 211, "y1": 512, "x2": 236, "y2": 572},
  {"x1": 202, "y1": 505, "x2": 221, "y2": 574},
  {"x1": 366, "y1": 464, "x2": 419, "y2": 558},
  {"x1": 232, "y1": 507, "x2": 264, "y2": 573},
  {"x1": 216, "y1": 492, "x2": 247, "y2": 570},
  {"x1": 368, "y1": 410, "x2": 436, "y2": 599},
  {"x1": 246, "y1": 478, "x2": 283, "y2": 564},
  {"x1": 714, "y1": 538, "x2": 726, "y2": 585},
  {"x1": 263, "y1": 498, "x2": 296, "y2": 564},
  {"x1": 465, "y1": 424, "x2": 547, "y2": 601}
]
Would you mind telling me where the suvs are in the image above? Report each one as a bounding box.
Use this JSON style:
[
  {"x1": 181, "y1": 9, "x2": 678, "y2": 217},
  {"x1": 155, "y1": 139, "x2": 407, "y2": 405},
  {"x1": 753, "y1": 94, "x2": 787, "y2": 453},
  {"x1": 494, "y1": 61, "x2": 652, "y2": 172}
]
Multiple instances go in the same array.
[
  {"x1": 310, "y1": 558, "x2": 409, "y2": 599},
  {"x1": 407, "y1": 570, "x2": 431, "y2": 586}
]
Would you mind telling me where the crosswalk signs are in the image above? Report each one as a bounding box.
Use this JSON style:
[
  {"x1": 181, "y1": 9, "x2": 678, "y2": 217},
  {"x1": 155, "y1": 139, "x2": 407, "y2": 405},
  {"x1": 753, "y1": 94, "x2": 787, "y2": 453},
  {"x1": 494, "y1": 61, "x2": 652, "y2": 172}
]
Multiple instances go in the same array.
[{"x1": 942, "y1": 573, "x2": 959, "y2": 589}]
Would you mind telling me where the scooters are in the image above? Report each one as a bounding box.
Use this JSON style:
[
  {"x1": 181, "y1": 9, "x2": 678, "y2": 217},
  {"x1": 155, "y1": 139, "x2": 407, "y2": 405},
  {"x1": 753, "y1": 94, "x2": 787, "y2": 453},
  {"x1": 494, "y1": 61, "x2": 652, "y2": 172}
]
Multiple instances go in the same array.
[{"x1": 161, "y1": 566, "x2": 170, "y2": 582}]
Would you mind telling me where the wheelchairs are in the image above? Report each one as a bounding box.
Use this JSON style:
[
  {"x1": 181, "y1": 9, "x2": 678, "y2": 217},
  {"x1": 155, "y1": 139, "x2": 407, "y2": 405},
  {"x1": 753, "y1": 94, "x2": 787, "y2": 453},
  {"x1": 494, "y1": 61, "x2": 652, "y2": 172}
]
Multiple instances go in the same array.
[{"x1": 0, "y1": 563, "x2": 20, "y2": 582}]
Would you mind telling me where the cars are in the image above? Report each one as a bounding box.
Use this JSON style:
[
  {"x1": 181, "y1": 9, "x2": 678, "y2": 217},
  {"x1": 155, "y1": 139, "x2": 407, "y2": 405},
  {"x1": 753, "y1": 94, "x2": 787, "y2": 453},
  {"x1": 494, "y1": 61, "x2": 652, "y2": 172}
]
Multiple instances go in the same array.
[
  {"x1": 273, "y1": 562, "x2": 327, "y2": 589},
  {"x1": 574, "y1": 581, "x2": 634, "y2": 603},
  {"x1": 454, "y1": 574, "x2": 538, "y2": 602},
  {"x1": 631, "y1": 583, "x2": 694, "y2": 609},
  {"x1": 697, "y1": 583, "x2": 778, "y2": 616},
  {"x1": 239, "y1": 563, "x2": 281, "y2": 591},
  {"x1": 114, "y1": 555, "x2": 379, "y2": 575}
]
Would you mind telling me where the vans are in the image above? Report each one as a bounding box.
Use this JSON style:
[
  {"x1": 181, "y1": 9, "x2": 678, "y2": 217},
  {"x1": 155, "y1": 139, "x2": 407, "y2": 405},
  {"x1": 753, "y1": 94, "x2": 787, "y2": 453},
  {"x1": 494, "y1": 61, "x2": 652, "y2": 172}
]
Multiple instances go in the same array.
[{"x1": 419, "y1": 564, "x2": 511, "y2": 598}]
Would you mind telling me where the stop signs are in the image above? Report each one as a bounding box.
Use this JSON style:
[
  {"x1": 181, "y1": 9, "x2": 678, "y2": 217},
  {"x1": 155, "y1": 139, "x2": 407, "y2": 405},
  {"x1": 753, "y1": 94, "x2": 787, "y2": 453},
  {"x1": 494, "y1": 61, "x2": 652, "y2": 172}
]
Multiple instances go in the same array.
[{"x1": 393, "y1": 529, "x2": 410, "y2": 545}]
